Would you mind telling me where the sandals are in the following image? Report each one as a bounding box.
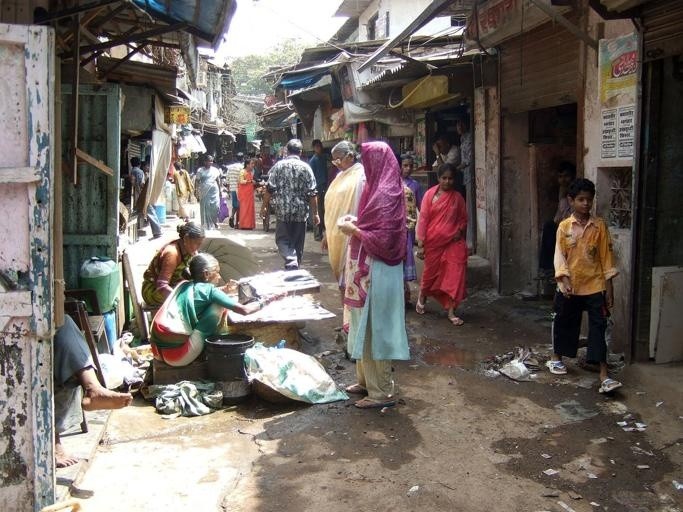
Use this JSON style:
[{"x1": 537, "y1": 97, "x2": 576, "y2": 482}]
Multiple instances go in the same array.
[
  {"x1": 600, "y1": 379, "x2": 622, "y2": 394},
  {"x1": 544, "y1": 359, "x2": 567, "y2": 374}
]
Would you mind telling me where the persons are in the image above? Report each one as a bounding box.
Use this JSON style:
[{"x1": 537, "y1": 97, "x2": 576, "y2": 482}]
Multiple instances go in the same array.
[
  {"x1": 141, "y1": 222, "x2": 287, "y2": 367},
  {"x1": 534, "y1": 163, "x2": 622, "y2": 393},
  {"x1": 320, "y1": 140, "x2": 469, "y2": 408},
  {"x1": 131, "y1": 138, "x2": 327, "y2": 271},
  {"x1": 55, "y1": 314, "x2": 132, "y2": 467}
]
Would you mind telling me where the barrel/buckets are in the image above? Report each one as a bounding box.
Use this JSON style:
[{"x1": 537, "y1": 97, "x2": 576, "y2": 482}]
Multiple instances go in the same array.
[
  {"x1": 78, "y1": 254, "x2": 121, "y2": 316},
  {"x1": 153, "y1": 204, "x2": 167, "y2": 224},
  {"x1": 203, "y1": 334, "x2": 254, "y2": 405}
]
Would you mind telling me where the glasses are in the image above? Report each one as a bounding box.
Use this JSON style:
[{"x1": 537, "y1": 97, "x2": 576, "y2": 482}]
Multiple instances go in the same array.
[{"x1": 329, "y1": 153, "x2": 351, "y2": 164}]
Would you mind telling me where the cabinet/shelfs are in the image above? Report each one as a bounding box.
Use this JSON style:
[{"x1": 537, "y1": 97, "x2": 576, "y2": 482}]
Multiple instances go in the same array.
[{"x1": 410, "y1": 168, "x2": 438, "y2": 196}]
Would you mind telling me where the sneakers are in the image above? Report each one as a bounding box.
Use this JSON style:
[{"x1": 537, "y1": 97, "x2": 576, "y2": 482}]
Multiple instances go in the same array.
[{"x1": 229, "y1": 217, "x2": 239, "y2": 229}]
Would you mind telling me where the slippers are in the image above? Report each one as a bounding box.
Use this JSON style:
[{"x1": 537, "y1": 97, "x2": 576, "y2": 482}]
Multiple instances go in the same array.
[
  {"x1": 353, "y1": 398, "x2": 398, "y2": 408},
  {"x1": 344, "y1": 383, "x2": 365, "y2": 392},
  {"x1": 415, "y1": 300, "x2": 426, "y2": 315},
  {"x1": 448, "y1": 318, "x2": 463, "y2": 324}
]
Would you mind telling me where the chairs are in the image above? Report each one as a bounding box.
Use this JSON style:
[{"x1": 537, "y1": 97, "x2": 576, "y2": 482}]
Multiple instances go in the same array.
[
  {"x1": 64, "y1": 288, "x2": 111, "y2": 389},
  {"x1": 54, "y1": 300, "x2": 88, "y2": 434}
]
